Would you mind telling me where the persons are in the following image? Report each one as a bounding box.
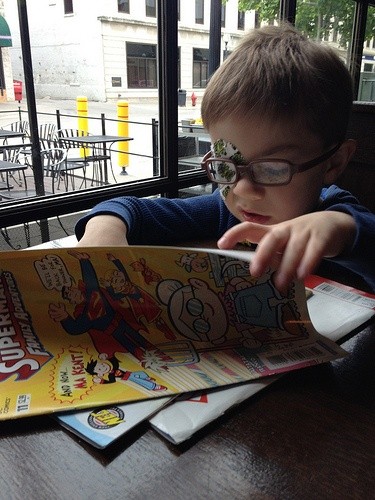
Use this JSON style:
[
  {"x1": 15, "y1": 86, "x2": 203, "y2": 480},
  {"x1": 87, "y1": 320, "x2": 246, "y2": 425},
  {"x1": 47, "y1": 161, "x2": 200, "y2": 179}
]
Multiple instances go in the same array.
[{"x1": 73, "y1": 26, "x2": 375, "y2": 292}]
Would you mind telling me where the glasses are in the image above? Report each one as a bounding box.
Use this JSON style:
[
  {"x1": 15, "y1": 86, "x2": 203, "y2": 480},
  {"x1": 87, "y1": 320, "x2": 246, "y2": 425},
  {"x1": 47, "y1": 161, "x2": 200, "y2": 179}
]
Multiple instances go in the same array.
[{"x1": 200, "y1": 146, "x2": 339, "y2": 187}]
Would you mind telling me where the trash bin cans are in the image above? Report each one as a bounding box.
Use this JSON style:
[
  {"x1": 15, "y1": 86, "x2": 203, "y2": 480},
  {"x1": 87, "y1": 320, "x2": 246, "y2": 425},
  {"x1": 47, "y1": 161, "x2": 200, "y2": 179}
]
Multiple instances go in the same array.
[{"x1": 178, "y1": 89, "x2": 186, "y2": 106}]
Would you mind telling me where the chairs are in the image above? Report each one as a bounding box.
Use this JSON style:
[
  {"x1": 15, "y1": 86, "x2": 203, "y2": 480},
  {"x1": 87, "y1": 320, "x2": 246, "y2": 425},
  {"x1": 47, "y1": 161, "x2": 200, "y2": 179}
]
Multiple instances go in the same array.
[
  {"x1": 0, "y1": 120, "x2": 103, "y2": 250},
  {"x1": 327, "y1": 101, "x2": 375, "y2": 215}
]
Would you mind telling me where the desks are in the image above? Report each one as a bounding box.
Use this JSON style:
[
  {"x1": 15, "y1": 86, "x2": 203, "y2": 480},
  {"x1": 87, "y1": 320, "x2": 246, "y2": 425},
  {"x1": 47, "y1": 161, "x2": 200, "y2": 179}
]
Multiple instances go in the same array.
[
  {"x1": 60, "y1": 135, "x2": 134, "y2": 190},
  {"x1": 1, "y1": 315, "x2": 375, "y2": 500}
]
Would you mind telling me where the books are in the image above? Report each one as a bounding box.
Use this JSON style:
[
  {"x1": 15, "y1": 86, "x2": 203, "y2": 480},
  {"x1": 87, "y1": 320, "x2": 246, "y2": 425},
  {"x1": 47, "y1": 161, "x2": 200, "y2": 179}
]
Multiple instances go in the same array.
[{"x1": 0, "y1": 246, "x2": 375, "y2": 449}]
